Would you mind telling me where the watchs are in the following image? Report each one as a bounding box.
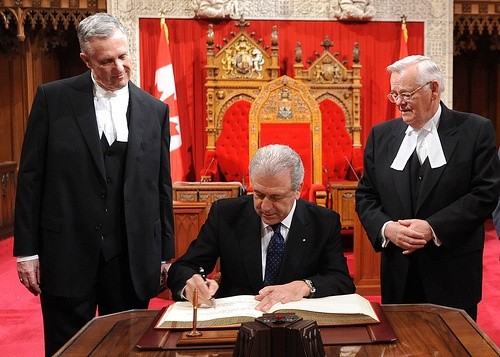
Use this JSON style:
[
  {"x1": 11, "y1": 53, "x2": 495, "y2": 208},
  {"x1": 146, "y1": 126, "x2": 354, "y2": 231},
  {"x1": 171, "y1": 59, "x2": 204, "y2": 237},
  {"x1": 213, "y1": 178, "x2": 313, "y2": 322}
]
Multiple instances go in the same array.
[{"x1": 303, "y1": 279, "x2": 316, "y2": 293}]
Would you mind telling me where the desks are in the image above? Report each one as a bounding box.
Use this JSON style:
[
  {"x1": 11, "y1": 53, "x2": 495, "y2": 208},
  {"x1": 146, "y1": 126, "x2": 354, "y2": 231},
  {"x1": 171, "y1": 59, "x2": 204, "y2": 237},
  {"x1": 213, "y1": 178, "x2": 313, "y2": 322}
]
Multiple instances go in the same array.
[{"x1": 50, "y1": 301, "x2": 500, "y2": 357}]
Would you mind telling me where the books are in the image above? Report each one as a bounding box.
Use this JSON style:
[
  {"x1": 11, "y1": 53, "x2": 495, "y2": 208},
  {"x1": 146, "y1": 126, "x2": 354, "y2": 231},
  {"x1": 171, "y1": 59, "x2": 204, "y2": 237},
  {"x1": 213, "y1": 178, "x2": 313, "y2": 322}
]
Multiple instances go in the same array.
[{"x1": 135, "y1": 295, "x2": 397, "y2": 349}]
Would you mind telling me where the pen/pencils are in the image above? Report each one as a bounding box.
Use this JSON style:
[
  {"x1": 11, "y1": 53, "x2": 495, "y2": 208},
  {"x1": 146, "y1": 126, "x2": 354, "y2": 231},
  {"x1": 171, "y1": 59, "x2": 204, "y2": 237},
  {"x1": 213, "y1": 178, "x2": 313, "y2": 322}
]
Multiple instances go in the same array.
[
  {"x1": 190, "y1": 288, "x2": 200, "y2": 333},
  {"x1": 199, "y1": 267, "x2": 215, "y2": 309}
]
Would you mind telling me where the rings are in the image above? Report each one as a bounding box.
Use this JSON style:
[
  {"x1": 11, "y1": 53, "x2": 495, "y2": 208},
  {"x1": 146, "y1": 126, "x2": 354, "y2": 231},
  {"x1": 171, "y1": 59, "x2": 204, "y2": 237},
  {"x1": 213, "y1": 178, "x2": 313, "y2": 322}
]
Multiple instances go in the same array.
[{"x1": 19, "y1": 279, "x2": 22, "y2": 281}]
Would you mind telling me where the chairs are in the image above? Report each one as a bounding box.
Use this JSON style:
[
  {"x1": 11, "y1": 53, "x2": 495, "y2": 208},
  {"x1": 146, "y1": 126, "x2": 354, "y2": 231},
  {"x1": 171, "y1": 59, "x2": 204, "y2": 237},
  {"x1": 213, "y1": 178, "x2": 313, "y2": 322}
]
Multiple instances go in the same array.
[
  {"x1": 246, "y1": 72, "x2": 330, "y2": 208},
  {"x1": 293, "y1": 34, "x2": 366, "y2": 186},
  {"x1": 199, "y1": 11, "x2": 281, "y2": 181}
]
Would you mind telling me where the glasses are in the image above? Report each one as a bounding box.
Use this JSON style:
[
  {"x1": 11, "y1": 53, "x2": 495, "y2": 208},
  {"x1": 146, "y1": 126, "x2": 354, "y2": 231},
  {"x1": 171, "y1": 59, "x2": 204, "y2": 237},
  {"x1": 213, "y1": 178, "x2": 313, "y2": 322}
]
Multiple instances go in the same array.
[{"x1": 387, "y1": 81, "x2": 432, "y2": 103}]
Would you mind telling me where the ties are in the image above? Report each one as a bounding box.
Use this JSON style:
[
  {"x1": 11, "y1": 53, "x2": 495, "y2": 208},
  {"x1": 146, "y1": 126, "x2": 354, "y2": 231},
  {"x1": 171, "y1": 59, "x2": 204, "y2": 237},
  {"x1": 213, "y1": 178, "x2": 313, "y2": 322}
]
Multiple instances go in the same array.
[{"x1": 263, "y1": 222, "x2": 285, "y2": 284}]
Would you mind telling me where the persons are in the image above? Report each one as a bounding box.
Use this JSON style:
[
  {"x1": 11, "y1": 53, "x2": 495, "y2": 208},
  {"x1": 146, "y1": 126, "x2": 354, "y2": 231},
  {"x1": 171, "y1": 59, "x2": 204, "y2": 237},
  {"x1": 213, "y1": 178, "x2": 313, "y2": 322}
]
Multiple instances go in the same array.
[
  {"x1": 166, "y1": 144, "x2": 357, "y2": 308},
  {"x1": 13, "y1": 12, "x2": 175, "y2": 357},
  {"x1": 354, "y1": 54, "x2": 500, "y2": 324}
]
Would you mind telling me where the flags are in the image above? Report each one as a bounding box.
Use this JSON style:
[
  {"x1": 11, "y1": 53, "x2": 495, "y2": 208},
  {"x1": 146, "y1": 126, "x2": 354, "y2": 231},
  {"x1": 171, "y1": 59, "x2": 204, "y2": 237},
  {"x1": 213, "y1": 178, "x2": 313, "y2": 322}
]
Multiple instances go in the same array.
[
  {"x1": 396, "y1": 20, "x2": 408, "y2": 117},
  {"x1": 154, "y1": 17, "x2": 186, "y2": 183}
]
